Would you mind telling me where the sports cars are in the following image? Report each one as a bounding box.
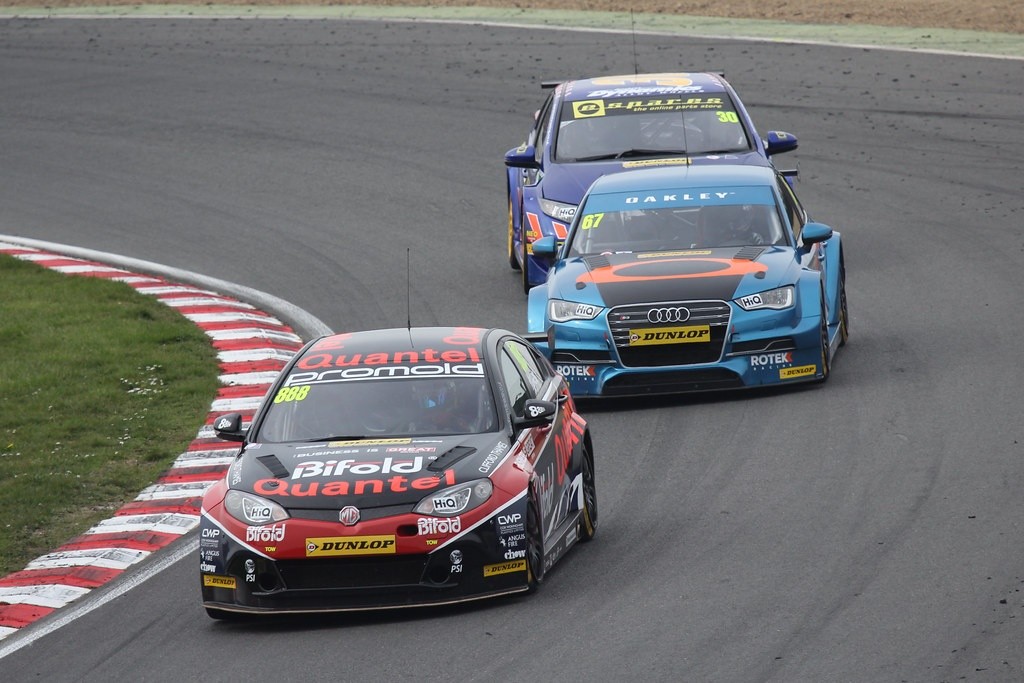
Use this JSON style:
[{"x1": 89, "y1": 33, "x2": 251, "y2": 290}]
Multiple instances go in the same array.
[
  {"x1": 503, "y1": 69, "x2": 802, "y2": 296},
  {"x1": 197, "y1": 326, "x2": 597, "y2": 621},
  {"x1": 525, "y1": 163, "x2": 851, "y2": 404}
]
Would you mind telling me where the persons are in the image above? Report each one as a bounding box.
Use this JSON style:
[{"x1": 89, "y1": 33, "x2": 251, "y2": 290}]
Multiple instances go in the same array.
[
  {"x1": 699, "y1": 202, "x2": 764, "y2": 249},
  {"x1": 673, "y1": 110, "x2": 742, "y2": 149},
  {"x1": 390, "y1": 379, "x2": 473, "y2": 434}
]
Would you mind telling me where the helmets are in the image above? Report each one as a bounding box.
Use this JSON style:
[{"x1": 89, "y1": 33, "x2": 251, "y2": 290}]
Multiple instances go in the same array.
[{"x1": 728, "y1": 205, "x2": 754, "y2": 232}]
